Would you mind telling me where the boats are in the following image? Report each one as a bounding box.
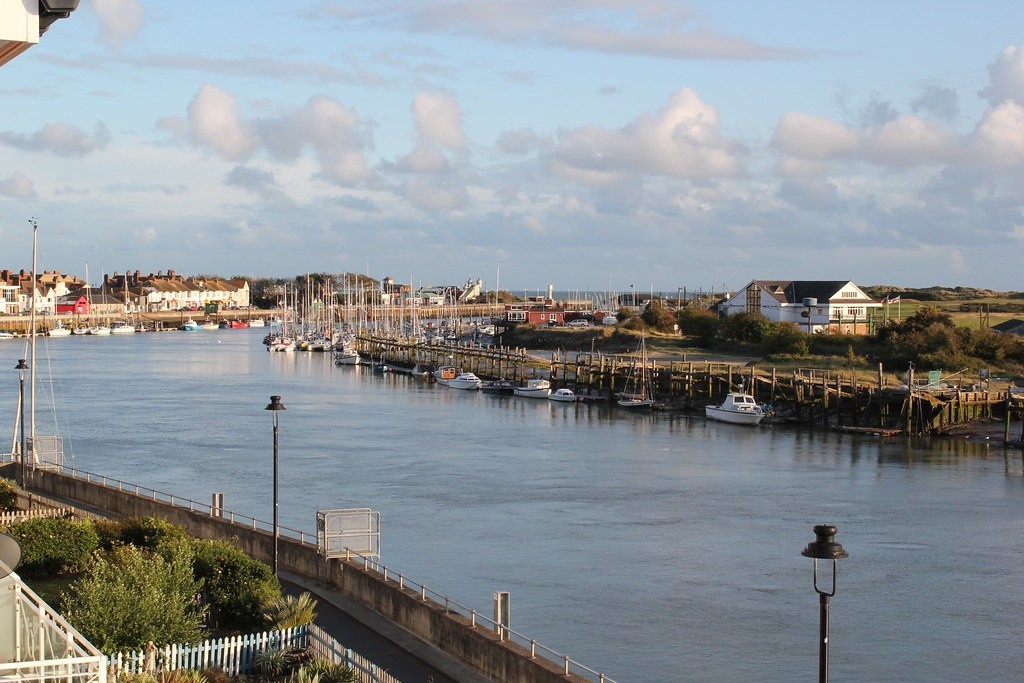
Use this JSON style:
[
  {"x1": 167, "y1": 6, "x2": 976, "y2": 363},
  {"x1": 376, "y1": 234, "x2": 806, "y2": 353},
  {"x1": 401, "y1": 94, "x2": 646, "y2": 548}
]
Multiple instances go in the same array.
[
  {"x1": 48, "y1": 329, "x2": 72, "y2": 337},
  {"x1": 411, "y1": 363, "x2": 437, "y2": 383},
  {"x1": 200, "y1": 315, "x2": 219, "y2": 331},
  {"x1": 432, "y1": 364, "x2": 462, "y2": 384},
  {"x1": 180, "y1": 317, "x2": 203, "y2": 331},
  {"x1": 335, "y1": 354, "x2": 357, "y2": 366},
  {"x1": 219, "y1": 319, "x2": 231, "y2": 329},
  {"x1": 231, "y1": 319, "x2": 249, "y2": 330},
  {"x1": 548, "y1": 389, "x2": 577, "y2": 403},
  {"x1": 448, "y1": 373, "x2": 482, "y2": 391},
  {"x1": 246, "y1": 319, "x2": 265, "y2": 328},
  {"x1": 513, "y1": 377, "x2": 552, "y2": 398},
  {"x1": 478, "y1": 377, "x2": 514, "y2": 395},
  {"x1": 704, "y1": 391, "x2": 766, "y2": 427},
  {"x1": 0, "y1": 333, "x2": 14, "y2": 340}
]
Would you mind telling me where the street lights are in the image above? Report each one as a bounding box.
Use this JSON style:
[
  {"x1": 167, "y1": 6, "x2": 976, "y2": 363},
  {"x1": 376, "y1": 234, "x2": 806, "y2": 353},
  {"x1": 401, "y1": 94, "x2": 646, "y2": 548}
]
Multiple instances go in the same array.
[
  {"x1": 631, "y1": 284, "x2": 636, "y2": 315},
  {"x1": 13, "y1": 359, "x2": 37, "y2": 492},
  {"x1": 677, "y1": 287, "x2": 682, "y2": 321},
  {"x1": 799, "y1": 518, "x2": 851, "y2": 683},
  {"x1": 262, "y1": 394, "x2": 290, "y2": 581},
  {"x1": 523, "y1": 288, "x2": 528, "y2": 310}
]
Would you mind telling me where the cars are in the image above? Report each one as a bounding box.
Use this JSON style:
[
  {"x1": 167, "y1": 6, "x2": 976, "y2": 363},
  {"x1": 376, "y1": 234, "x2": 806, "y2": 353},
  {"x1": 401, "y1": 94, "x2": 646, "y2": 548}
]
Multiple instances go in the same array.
[
  {"x1": 39, "y1": 311, "x2": 51, "y2": 316},
  {"x1": 65, "y1": 310, "x2": 73, "y2": 315},
  {"x1": 223, "y1": 305, "x2": 260, "y2": 310},
  {"x1": 176, "y1": 306, "x2": 192, "y2": 311},
  {"x1": 565, "y1": 318, "x2": 589, "y2": 327},
  {"x1": 537, "y1": 320, "x2": 559, "y2": 328}
]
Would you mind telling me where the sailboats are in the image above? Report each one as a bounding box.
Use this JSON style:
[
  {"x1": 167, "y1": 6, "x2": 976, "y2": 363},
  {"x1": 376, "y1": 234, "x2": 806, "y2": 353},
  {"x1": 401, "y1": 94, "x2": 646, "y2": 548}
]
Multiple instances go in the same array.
[
  {"x1": 602, "y1": 272, "x2": 618, "y2": 326},
  {"x1": 110, "y1": 272, "x2": 136, "y2": 334},
  {"x1": 72, "y1": 262, "x2": 93, "y2": 335},
  {"x1": 263, "y1": 269, "x2": 501, "y2": 355},
  {"x1": 617, "y1": 326, "x2": 657, "y2": 407},
  {"x1": 88, "y1": 265, "x2": 112, "y2": 335}
]
